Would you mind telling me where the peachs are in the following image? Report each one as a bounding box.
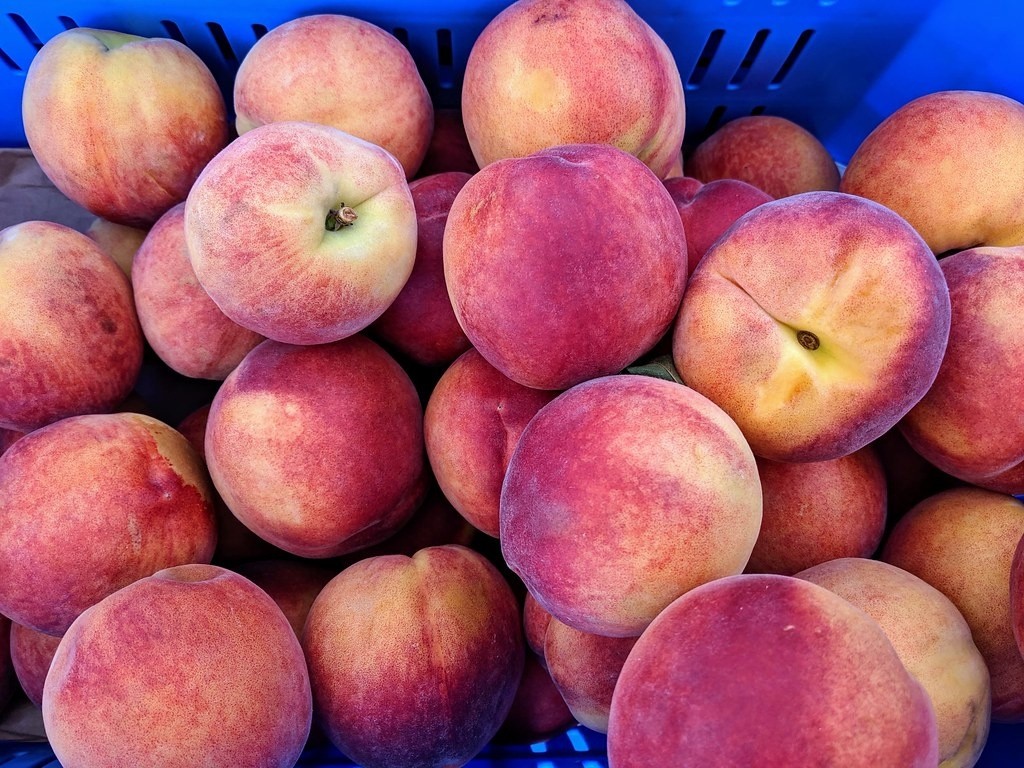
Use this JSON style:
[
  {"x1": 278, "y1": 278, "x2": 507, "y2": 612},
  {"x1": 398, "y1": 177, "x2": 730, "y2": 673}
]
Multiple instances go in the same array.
[{"x1": 0, "y1": 0, "x2": 1024, "y2": 768}]
